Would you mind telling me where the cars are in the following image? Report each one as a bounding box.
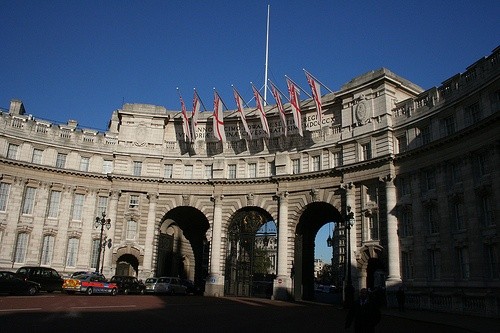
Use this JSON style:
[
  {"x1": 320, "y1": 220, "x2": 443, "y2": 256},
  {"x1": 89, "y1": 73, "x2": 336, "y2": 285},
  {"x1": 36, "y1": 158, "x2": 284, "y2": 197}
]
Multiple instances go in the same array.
[
  {"x1": 0, "y1": 271, "x2": 42, "y2": 296},
  {"x1": 61, "y1": 273, "x2": 119, "y2": 296},
  {"x1": 110, "y1": 276, "x2": 146, "y2": 295},
  {"x1": 15, "y1": 266, "x2": 65, "y2": 294},
  {"x1": 145, "y1": 276, "x2": 188, "y2": 295}
]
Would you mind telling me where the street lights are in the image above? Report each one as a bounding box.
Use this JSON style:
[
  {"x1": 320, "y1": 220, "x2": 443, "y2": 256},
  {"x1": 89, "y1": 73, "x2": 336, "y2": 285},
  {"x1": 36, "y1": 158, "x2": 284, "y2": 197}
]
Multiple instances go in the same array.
[
  {"x1": 341, "y1": 204, "x2": 357, "y2": 328},
  {"x1": 94, "y1": 211, "x2": 112, "y2": 272}
]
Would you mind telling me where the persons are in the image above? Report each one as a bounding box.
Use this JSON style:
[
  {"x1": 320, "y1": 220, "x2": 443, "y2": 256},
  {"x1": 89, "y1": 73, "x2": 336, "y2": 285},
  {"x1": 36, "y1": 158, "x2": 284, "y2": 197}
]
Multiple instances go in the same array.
[
  {"x1": 396, "y1": 288, "x2": 405, "y2": 312},
  {"x1": 333, "y1": 286, "x2": 342, "y2": 305},
  {"x1": 367, "y1": 287, "x2": 387, "y2": 312},
  {"x1": 345, "y1": 289, "x2": 382, "y2": 333}
]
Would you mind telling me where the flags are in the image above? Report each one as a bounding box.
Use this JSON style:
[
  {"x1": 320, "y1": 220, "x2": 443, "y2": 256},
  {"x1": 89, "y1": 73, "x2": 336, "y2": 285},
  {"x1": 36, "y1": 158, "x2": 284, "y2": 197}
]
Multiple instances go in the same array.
[
  {"x1": 286, "y1": 77, "x2": 301, "y2": 129},
  {"x1": 191, "y1": 91, "x2": 200, "y2": 139},
  {"x1": 233, "y1": 88, "x2": 250, "y2": 132},
  {"x1": 252, "y1": 86, "x2": 269, "y2": 133},
  {"x1": 305, "y1": 72, "x2": 322, "y2": 122},
  {"x1": 180, "y1": 96, "x2": 191, "y2": 142},
  {"x1": 270, "y1": 82, "x2": 287, "y2": 127},
  {"x1": 213, "y1": 91, "x2": 226, "y2": 143}
]
